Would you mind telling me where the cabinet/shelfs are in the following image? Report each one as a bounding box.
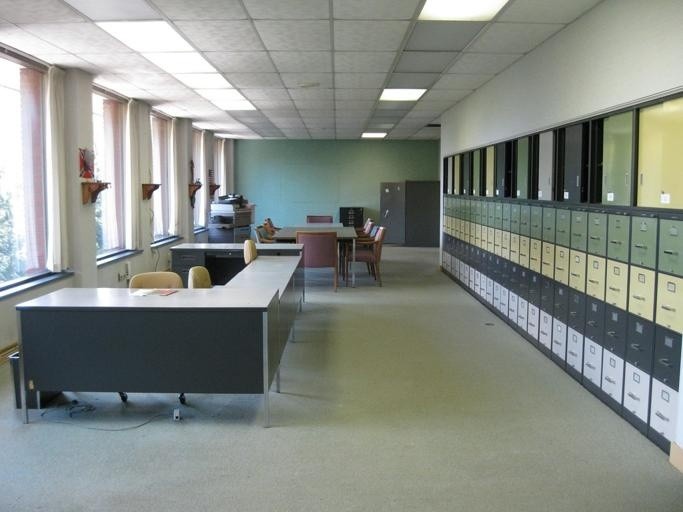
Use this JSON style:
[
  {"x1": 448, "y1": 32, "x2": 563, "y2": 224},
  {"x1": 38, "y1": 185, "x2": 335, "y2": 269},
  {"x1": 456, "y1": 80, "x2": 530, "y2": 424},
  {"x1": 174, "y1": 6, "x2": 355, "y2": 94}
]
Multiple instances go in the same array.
[{"x1": 442, "y1": 86, "x2": 683, "y2": 210}]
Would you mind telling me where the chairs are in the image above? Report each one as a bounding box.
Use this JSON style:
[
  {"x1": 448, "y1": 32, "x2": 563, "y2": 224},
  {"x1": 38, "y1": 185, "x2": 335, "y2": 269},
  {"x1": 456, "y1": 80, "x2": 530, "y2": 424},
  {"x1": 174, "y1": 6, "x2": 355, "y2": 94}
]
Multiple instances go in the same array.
[
  {"x1": 244, "y1": 240, "x2": 257, "y2": 264},
  {"x1": 344, "y1": 218, "x2": 387, "y2": 287},
  {"x1": 297, "y1": 231, "x2": 338, "y2": 289},
  {"x1": 188, "y1": 266, "x2": 211, "y2": 288},
  {"x1": 306, "y1": 216, "x2": 333, "y2": 224},
  {"x1": 130, "y1": 272, "x2": 183, "y2": 288},
  {"x1": 255, "y1": 217, "x2": 281, "y2": 243}
]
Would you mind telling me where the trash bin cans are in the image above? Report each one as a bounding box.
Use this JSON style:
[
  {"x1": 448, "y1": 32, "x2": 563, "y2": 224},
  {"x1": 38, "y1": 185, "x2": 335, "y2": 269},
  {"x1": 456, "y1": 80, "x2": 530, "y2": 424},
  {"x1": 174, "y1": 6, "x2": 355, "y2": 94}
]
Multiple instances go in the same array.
[{"x1": 8, "y1": 351, "x2": 61, "y2": 410}]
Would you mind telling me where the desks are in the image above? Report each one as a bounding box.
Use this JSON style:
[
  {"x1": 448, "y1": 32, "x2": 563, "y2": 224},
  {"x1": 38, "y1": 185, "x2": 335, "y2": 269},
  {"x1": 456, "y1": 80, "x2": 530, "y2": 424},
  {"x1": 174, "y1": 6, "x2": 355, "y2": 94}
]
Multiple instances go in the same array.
[{"x1": 272, "y1": 225, "x2": 358, "y2": 238}]
